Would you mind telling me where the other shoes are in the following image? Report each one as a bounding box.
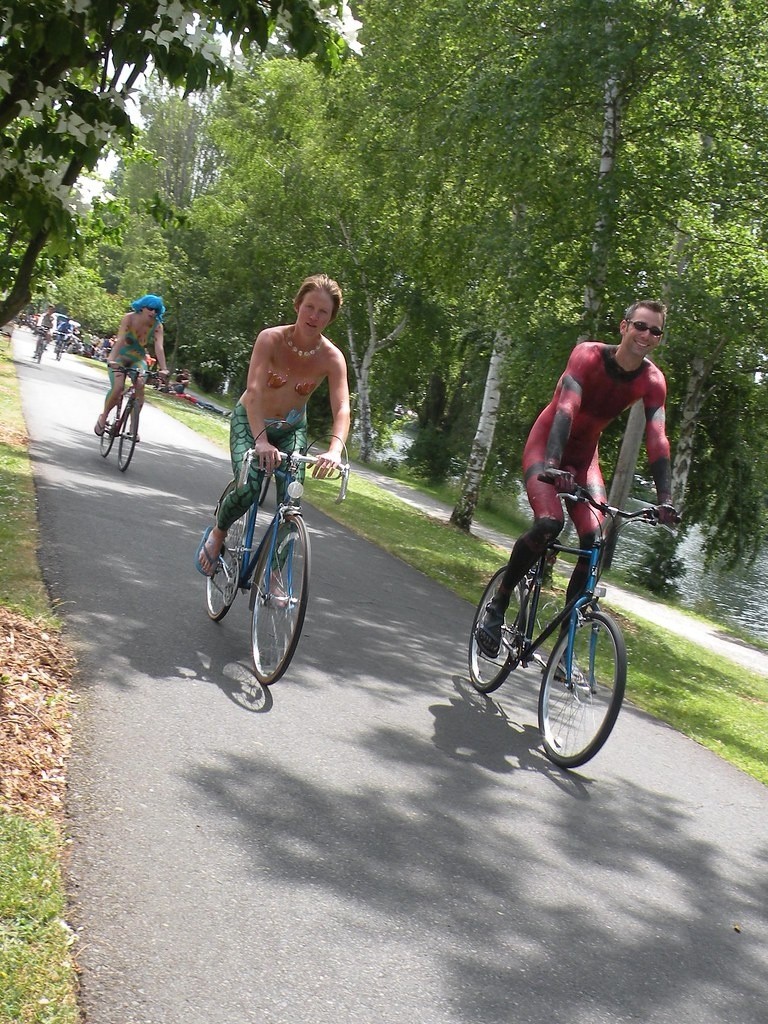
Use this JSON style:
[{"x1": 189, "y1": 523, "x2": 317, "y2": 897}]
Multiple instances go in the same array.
[{"x1": 222, "y1": 411, "x2": 232, "y2": 416}]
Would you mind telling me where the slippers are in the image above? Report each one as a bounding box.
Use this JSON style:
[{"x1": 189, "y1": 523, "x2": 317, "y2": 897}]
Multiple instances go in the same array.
[
  {"x1": 266, "y1": 585, "x2": 296, "y2": 611},
  {"x1": 94, "y1": 414, "x2": 106, "y2": 436},
  {"x1": 127, "y1": 431, "x2": 142, "y2": 443},
  {"x1": 195, "y1": 525, "x2": 221, "y2": 578}
]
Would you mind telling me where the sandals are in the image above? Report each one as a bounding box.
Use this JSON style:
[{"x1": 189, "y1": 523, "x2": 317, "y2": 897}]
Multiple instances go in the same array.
[
  {"x1": 557, "y1": 655, "x2": 585, "y2": 686},
  {"x1": 476, "y1": 601, "x2": 505, "y2": 660}
]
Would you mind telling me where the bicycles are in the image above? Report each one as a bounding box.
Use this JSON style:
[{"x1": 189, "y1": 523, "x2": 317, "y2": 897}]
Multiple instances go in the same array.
[
  {"x1": 465, "y1": 473, "x2": 683, "y2": 770},
  {"x1": 203, "y1": 419, "x2": 352, "y2": 687},
  {"x1": 33, "y1": 325, "x2": 75, "y2": 365},
  {"x1": 99, "y1": 363, "x2": 171, "y2": 473}
]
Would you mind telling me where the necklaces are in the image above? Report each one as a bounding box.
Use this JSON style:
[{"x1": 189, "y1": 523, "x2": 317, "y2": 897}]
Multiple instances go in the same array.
[{"x1": 287, "y1": 333, "x2": 324, "y2": 356}]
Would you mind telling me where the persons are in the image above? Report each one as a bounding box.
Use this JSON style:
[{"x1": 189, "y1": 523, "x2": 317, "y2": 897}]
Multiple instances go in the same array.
[
  {"x1": 195, "y1": 273, "x2": 350, "y2": 608},
  {"x1": 94, "y1": 295, "x2": 169, "y2": 441},
  {"x1": 19, "y1": 303, "x2": 232, "y2": 415},
  {"x1": 476, "y1": 300, "x2": 676, "y2": 693}
]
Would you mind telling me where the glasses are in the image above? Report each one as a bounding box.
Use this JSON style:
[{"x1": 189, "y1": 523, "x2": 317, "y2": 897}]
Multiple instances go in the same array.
[
  {"x1": 147, "y1": 307, "x2": 160, "y2": 314},
  {"x1": 626, "y1": 318, "x2": 664, "y2": 336}
]
